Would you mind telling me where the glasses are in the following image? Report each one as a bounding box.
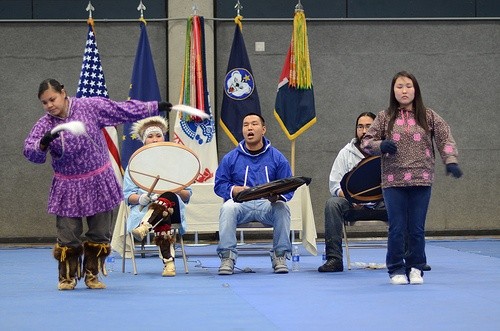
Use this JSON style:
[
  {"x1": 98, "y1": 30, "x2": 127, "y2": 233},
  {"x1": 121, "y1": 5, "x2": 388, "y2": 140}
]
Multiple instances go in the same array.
[{"x1": 356, "y1": 125, "x2": 372, "y2": 131}]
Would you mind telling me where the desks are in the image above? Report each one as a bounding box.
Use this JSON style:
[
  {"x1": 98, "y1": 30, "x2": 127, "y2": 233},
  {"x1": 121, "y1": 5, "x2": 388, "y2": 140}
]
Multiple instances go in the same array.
[{"x1": 111, "y1": 183, "x2": 317, "y2": 258}]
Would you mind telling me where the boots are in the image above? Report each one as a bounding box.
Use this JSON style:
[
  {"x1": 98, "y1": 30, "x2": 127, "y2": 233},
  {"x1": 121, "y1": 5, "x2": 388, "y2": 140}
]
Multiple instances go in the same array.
[
  {"x1": 83, "y1": 241, "x2": 112, "y2": 289},
  {"x1": 53, "y1": 244, "x2": 84, "y2": 290}
]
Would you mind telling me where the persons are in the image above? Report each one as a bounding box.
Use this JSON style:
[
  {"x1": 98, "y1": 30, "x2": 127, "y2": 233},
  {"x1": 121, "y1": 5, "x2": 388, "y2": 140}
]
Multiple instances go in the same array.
[
  {"x1": 24, "y1": 78, "x2": 173, "y2": 288},
  {"x1": 360, "y1": 71, "x2": 464, "y2": 285},
  {"x1": 318, "y1": 112, "x2": 431, "y2": 272},
  {"x1": 122, "y1": 115, "x2": 192, "y2": 276},
  {"x1": 214, "y1": 113, "x2": 295, "y2": 274}
]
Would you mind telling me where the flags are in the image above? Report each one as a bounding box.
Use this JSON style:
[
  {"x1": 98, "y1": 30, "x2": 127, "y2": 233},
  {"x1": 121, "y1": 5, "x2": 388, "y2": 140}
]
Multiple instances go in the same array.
[
  {"x1": 120, "y1": 19, "x2": 169, "y2": 176},
  {"x1": 273, "y1": 12, "x2": 316, "y2": 140},
  {"x1": 173, "y1": 15, "x2": 218, "y2": 183},
  {"x1": 219, "y1": 16, "x2": 260, "y2": 147},
  {"x1": 76, "y1": 18, "x2": 123, "y2": 188}
]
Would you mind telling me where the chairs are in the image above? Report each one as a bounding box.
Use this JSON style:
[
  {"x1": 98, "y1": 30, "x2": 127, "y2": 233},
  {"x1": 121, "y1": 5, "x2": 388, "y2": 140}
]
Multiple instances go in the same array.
[
  {"x1": 122, "y1": 207, "x2": 189, "y2": 276},
  {"x1": 343, "y1": 207, "x2": 388, "y2": 269},
  {"x1": 235, "y1": 220, "x2": 276, "y2": 233}
]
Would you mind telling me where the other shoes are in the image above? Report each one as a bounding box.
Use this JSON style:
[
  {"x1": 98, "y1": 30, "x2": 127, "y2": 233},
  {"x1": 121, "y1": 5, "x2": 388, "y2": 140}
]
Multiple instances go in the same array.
[
  {"x1": 131, "y1": 221, "x2": 149, "y2": 242},
  {"x1": 161, "y1": 261, "x2": 177, "y2": 277}
]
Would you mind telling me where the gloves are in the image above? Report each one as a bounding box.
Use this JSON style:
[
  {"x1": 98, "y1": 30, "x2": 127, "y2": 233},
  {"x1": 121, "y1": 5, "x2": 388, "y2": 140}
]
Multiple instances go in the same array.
[
  {"x1": 158, "y1": 101, "x2": 173, "y2": 112},
  {"x1": 40, "y1": 131, "x2": 60, "y2": 147},
  {"x1": 446, "y1": 163, "x2": 463, "y2": 178},
  {"x1": 380, "y1": 140, "x2": 398, "y2": 156},
  {"x1": 138, "y1": 194, "x2": 151, "y2": 206}
]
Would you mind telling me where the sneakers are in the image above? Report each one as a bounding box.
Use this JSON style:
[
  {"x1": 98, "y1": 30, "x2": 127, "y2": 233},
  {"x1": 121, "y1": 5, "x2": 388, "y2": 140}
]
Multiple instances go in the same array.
[
  {"x1": 272, "y1": 256, "x2": 289, "y2": 273},
  {"x1": 390, "y1": 274, "x2": 409, "y2": 286},
  {"x1": 422, "y1": 264, "x2": 432, "y2": 271},
  {"x1": 318, "y1": 257, "x2": 344, "y2": 273},
  {"x1": 217, "y1": 258, "x2": 234, "y2": 275},
  {"x1": 409, "y1": 268, "x2": 424, "y2": 284}
]
